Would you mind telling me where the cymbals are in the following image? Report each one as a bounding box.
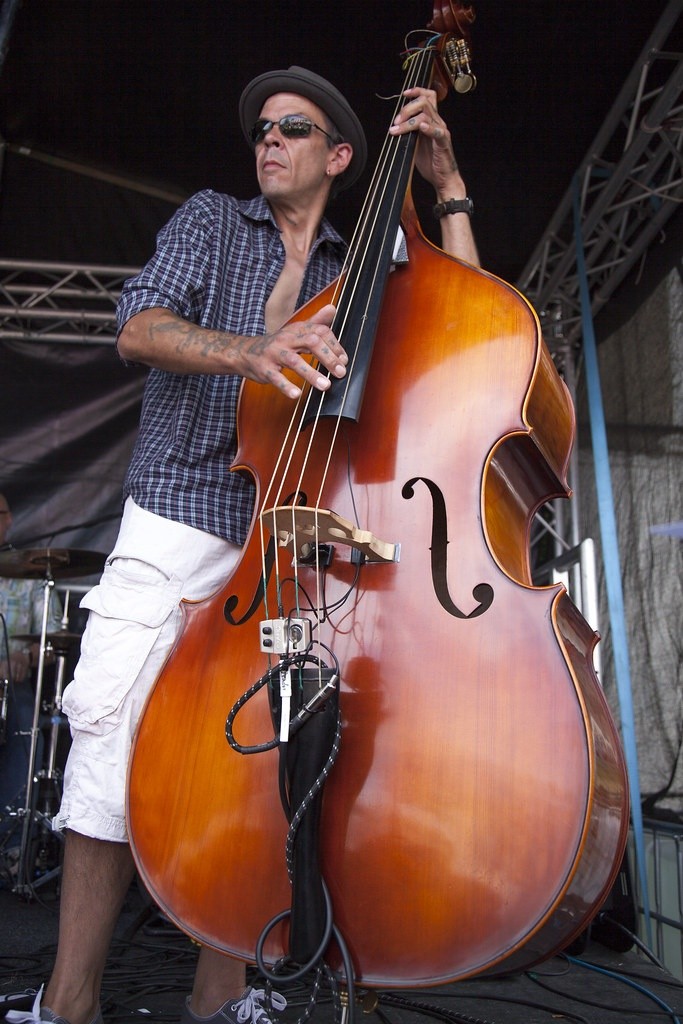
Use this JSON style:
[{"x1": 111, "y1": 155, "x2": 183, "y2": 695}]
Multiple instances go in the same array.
[
  {"x1": 0, "y1": 546, "x2": 109, "y2": 580},
  {"x1": 54, "y1": 583, "x2": 94, "y2": 593},
  {"x1": 10, "y1": 629, "x2": 82, "y2": 646}
]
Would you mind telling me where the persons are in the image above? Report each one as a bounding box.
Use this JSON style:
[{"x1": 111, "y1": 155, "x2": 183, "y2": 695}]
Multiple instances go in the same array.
[{"x1": 0, "y1": 66, "x2": 479, "y2": 1024}]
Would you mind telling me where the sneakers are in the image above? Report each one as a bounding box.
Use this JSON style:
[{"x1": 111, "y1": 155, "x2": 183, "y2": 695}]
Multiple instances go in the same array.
[
  {"x1": 180, "y1": 985, "x2": 286, "y2": 1024},
  {"x1": 3, "y1": 982, "x2": 105, "y2": 1024}
]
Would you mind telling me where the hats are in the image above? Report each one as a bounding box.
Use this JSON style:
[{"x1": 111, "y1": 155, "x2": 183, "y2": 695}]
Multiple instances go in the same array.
[{"x1": 239, "y1": 64, "x2": 368, "y2": 193}]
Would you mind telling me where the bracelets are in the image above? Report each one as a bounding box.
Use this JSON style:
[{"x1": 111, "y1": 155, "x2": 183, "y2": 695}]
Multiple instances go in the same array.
[{"x1": 434, "y1": 196, "x2": 474, "y2": 219}]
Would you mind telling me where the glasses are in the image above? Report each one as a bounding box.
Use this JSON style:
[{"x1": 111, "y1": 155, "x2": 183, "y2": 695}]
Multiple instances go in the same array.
[{"x1": 250, "y1": 113, "x2": 338, "y2": 150}]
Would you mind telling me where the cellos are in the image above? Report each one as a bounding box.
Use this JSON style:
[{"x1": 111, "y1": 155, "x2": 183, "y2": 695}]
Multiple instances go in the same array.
[{"x1": 112, "y1": 0, "x2": 637, "y2": 1006}]
